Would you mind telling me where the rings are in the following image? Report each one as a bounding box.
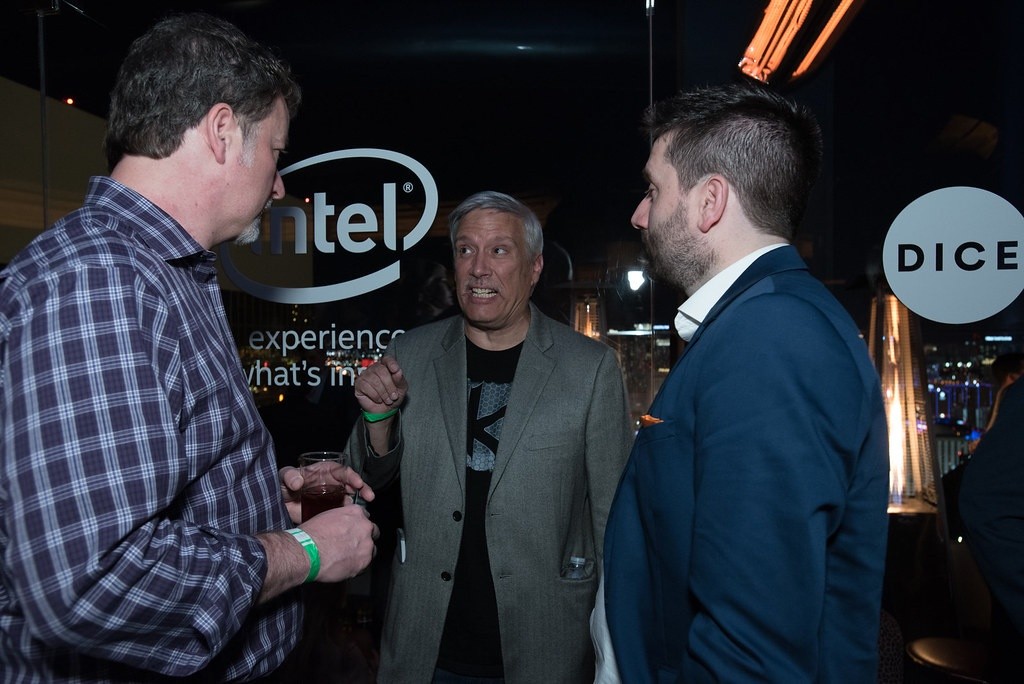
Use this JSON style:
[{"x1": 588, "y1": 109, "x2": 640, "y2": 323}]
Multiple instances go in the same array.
[{"x1": 322, "y1": 483, "x2": 326, "y2": 496}]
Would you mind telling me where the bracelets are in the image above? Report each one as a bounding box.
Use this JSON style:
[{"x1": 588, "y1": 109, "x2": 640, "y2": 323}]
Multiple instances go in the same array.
[
  {"x1": 283, "y1": 528, "x2": 321, "y2": 581},
  {"x1": 361, "y1": 408, "x2": 399, "y2": 421}
]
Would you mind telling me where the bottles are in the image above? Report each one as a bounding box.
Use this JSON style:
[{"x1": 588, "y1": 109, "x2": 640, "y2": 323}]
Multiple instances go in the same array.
[{"x1": 562, "y1": 556, "x2": 588, "y2": 578}]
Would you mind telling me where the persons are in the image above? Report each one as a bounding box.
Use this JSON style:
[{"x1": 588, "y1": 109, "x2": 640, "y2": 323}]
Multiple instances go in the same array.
[
  {"x1": 1, "y1": 15, "x2": 379, "y2": 684},
  {"x1": 587, "y1": 85, "x2": 890, "y2": 684},
  {"x1": 903, "y1": 350, "x2": 1024, "y2": 684},
  {"x1": 333, "y1": 190, "x2": 635, "y2": 684}
]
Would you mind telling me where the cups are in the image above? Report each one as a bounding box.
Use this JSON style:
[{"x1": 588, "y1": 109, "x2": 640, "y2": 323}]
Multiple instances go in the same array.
[{"x1": 301, "y1": 451, "x2": 349, "y2": 523}]
